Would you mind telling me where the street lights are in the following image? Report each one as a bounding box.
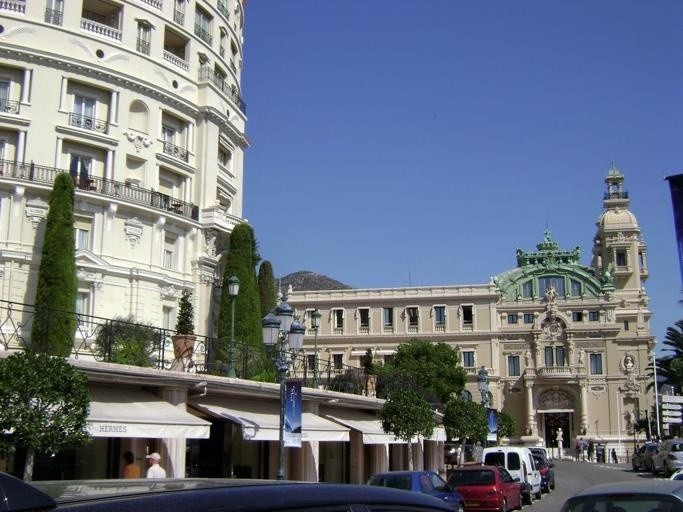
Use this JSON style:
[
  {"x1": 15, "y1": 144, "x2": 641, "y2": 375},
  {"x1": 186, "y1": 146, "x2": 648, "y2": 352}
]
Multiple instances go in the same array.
[
  {"x1": 477, "y1": 366, "x2": 492, "y2": 446},
  {"x1": 225, "y1": 271, "x2": 241, "y2": 379},
  {"x1": 311, "y1": 306, "x2": 322, "y2": 388},
  {"x1": 648, "y1": 351, "x2": 663, "y2": 440},
  {"x1": 262, "y1": 292, "x2": 306, "y2": 482}
]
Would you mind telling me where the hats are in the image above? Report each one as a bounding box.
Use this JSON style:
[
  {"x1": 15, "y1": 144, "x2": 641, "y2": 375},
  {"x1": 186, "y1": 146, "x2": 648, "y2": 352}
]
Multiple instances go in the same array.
[{"x1": 146, "y1": 452, "x2": 161, "y2": 460}]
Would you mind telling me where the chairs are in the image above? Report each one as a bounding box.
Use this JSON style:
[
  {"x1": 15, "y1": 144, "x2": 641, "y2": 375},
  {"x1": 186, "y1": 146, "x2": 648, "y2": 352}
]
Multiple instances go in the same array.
[
  {"x1": 612, "y1": 507, "x2": 626, "y2": 512},
  {"x1": 648, "y1": 509, "x2": 660, "y2": 512}
]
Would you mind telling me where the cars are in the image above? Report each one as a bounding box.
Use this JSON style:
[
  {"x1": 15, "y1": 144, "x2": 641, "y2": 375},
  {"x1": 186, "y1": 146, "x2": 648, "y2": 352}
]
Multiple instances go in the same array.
[
  {"x1": 561, "y1": 480, "x2": 683, "y2": 511},
  {"x1": 670, "y1": 469, "x2": 682, "y2": 483},
  {"x1": 651, "y1": 439, "x2": 683, "y2": 475},
  {"x1": 632, "y1": 443, "x2": 658, "y2": 472},
  {"x1": 0, "y1": 467, "x2": 462, "y2": 511},
  {"x1": 369, "y1": 444, "x2": 557, "y2": 512}
]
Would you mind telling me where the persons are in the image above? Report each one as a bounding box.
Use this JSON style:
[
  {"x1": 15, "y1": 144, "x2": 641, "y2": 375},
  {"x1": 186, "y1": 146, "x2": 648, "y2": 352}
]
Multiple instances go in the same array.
[
  {"x1": 472, "y1": 441, "x2": 484, "y2": 462},
  {"x1": 120, "y1": 451, "x2": 141, "y2": 478},
  {"x1": 575, "y1": 438, "x2": 601, "y2": 463},
  {"x1": 146, "y1": 452, "x2": 167, "y2": 479},
  {"x1": 457, "y1": 444, "x2": 461, "y2": 466},
  {"x1": 625, "y1": 356, "x2": 633, "y2": 368},
  {"x1": 627, "y1": 409, "x2": 636, "y2": 424},
  {"x1": 478, "y1": 366, "x2": 489, "y2": 376},
  {"x1": 611, "y1": 448, "x2": 617, "y2": 464},
  {"x1": 578, "y1": 347, "x2": 585, "y2": 366},
  {"x1": 546, "y1": 286, "x2": 558, "y2": 302},
  {"x1": 524, "y1": 349, "x2": 531, "y2": 367}
]
